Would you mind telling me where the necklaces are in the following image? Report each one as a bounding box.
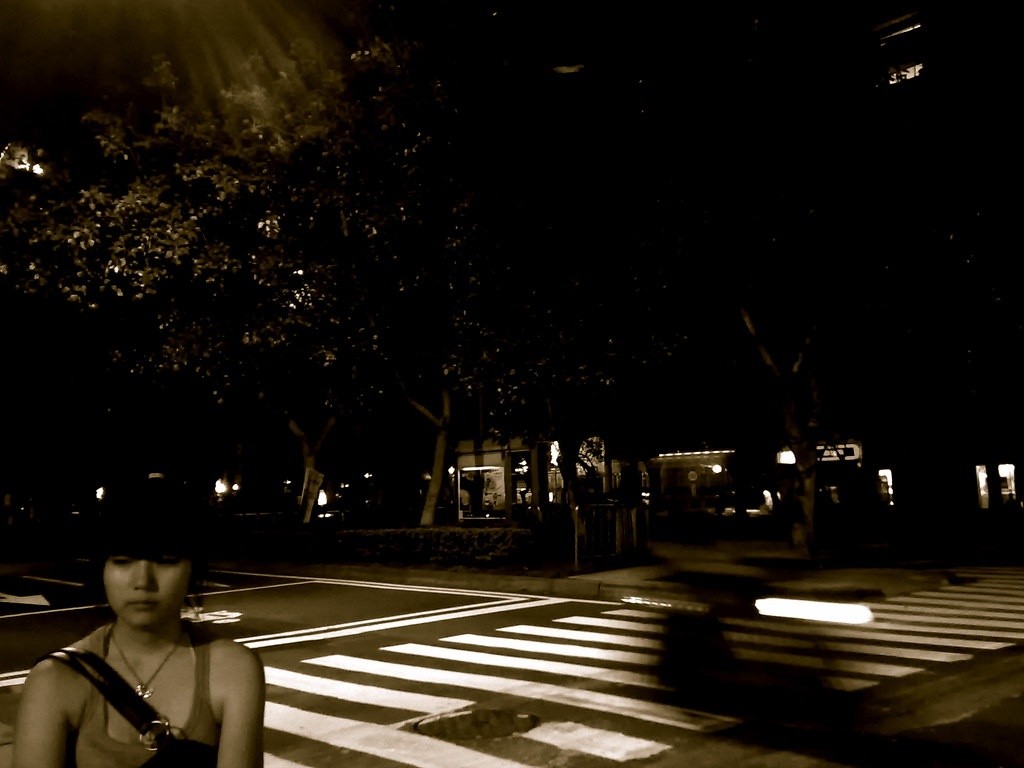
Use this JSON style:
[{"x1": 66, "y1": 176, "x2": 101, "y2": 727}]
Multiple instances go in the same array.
[{"x1": 112, "y1": 632, "x2": 180, "y2": 699}]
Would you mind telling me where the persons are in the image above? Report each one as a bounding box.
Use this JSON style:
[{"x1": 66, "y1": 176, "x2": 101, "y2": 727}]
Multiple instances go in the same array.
[{"x1": 12, "y1": 502, "x2": 267, "y2": 768}]
